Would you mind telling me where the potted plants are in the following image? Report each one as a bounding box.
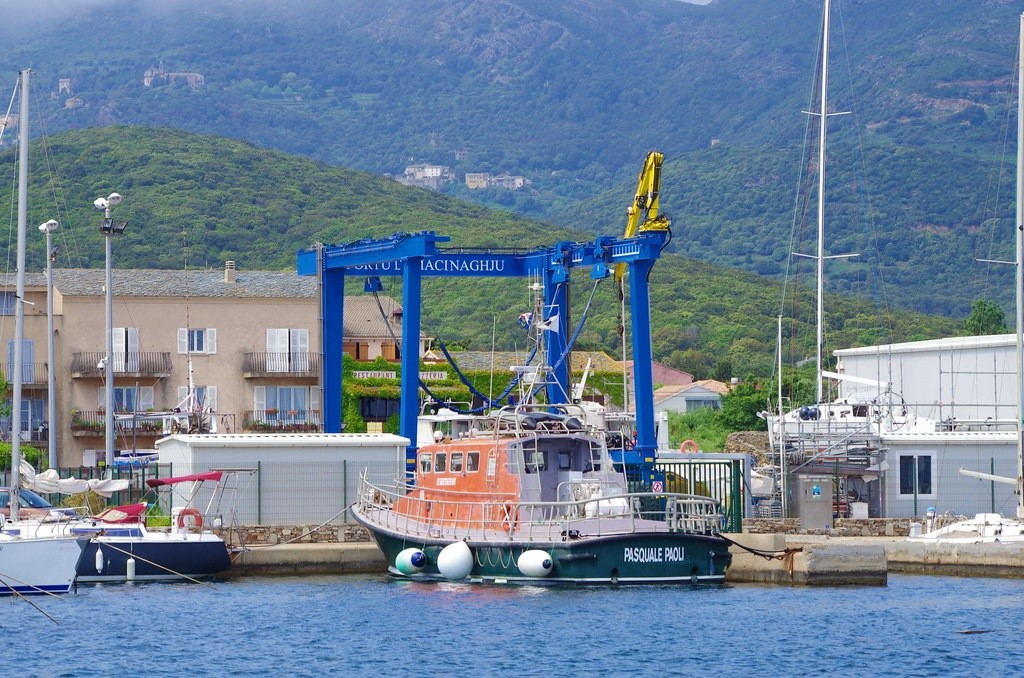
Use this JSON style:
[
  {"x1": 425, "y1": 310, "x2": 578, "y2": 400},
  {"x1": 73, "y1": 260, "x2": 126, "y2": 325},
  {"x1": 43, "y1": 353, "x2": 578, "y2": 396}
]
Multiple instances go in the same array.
[
  {"x1": 254, "y1": 420, "x2": 319, "y2": 432},
  {"x1": 70, "y1": 416, "x2": 163, "y2": 432}
]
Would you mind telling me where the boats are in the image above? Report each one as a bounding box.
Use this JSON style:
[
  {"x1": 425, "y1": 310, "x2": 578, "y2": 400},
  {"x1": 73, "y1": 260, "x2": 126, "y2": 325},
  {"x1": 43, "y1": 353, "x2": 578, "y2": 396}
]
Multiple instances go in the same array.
[
  {"x1": 70, "y1": 465, "x2": 245, "y2": 589},
  {"x1": 349, "y1": 266, "x2": 735, "y2": 588}
]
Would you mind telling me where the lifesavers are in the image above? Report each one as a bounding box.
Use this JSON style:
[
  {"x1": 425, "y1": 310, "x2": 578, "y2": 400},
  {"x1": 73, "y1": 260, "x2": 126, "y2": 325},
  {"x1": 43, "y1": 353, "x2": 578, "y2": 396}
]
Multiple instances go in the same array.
[
  {"x1": 500, "y1": 499, "x2": 517, "y2": 531},
  {"x1": 680, "y1": 440, "x2": 698, "y2": 453},
  {"x1": 177, "y1": 509, "x2": 202, "y2": 529}
]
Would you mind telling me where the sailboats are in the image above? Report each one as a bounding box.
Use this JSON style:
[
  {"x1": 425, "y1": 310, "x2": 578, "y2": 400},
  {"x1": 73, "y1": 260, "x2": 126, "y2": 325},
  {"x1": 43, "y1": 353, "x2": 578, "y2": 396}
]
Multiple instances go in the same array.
[
  {"x1": 0, "y1": 65, "x2": 83, "y2": 592},
  {"x1": 908, "y1": 10, "x2": 1024, "y2": 541},
  {"x1": 751, "y1": 0, "x2": 916, "y2": 462}
]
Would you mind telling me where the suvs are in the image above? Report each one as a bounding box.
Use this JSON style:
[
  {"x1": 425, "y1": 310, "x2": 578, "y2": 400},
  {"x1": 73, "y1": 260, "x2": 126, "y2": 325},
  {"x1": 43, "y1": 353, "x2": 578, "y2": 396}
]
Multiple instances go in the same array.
[{"x1": 0, "y1": 487, "x2": 81, "y2": 523}]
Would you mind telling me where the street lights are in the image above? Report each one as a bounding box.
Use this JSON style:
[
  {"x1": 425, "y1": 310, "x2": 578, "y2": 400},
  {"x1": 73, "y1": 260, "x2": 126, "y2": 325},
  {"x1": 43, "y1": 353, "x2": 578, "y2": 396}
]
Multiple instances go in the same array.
[
  {"x1": 92, "y1": 191, "x2": 128, "y2": 504},
  {"x1": 38, "y1": 219, "x2": 56, "y2": 470}
]
